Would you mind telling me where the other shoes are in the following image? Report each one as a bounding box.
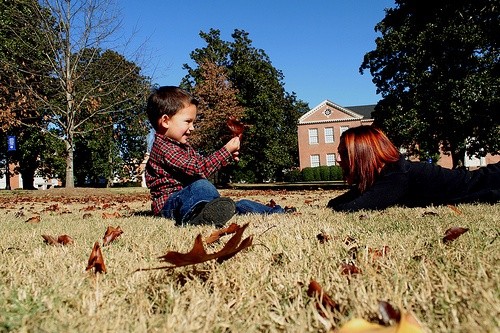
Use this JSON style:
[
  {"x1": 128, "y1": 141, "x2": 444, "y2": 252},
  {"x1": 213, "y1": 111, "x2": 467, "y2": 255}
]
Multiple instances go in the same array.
[{"x1": 190, "y1": 197, "x2": 237, "y2": 227}]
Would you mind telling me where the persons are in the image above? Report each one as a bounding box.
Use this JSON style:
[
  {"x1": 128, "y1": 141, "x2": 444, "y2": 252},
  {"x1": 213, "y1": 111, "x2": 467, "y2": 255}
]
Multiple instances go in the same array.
[
  {"x1": 327, "y1": 124, "x2": 500, "y2": 212},
  {"x1": 143, "y1": 85, "x2": 296, "y2": 225}
]
